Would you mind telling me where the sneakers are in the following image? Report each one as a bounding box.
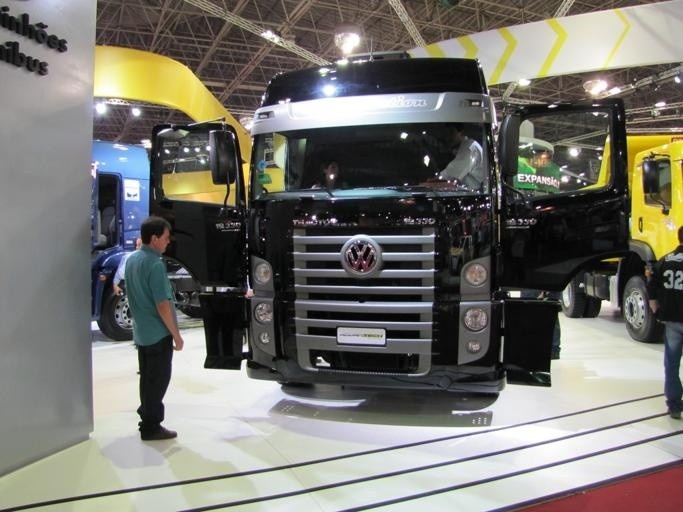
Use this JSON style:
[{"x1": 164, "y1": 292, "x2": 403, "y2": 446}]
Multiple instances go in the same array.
[{"x1": 141, "y1": 425, "x2": 178, "y2": 441}]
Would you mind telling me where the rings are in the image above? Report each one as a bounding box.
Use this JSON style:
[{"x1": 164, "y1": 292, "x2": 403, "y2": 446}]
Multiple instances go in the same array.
[{"x1": 178, "y1": 342, "x2": 181, "y2": 345}]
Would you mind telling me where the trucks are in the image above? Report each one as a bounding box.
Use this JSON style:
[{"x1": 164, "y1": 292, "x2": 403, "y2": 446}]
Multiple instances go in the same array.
[
  {"x1": 91, "y1": 46, "x2": 285, "y2": 342},
  {"x1": 559, "y1": 133, "x2": 683, "y2": 344},
  {"x1": 147, "y1": 50, "x2": 630, "y2": 399}
]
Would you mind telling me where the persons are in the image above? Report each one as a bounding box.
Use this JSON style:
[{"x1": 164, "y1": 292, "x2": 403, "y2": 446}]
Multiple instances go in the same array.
[
  {"x1": 124, "y1": 217, "x2": 183, "y2": 440},
  {"x1": 113, "y1": 237, "x2": 142, "y2": 374},
  {"x1": 427, "y1": 123, "x2": 482, "y2": 188},
  {"x1": 311, "y1": 159, "x2": 347, "y2": 190},
  {"x1": 647, "y1": 224, "x2": 682, "y2": 419}
]
